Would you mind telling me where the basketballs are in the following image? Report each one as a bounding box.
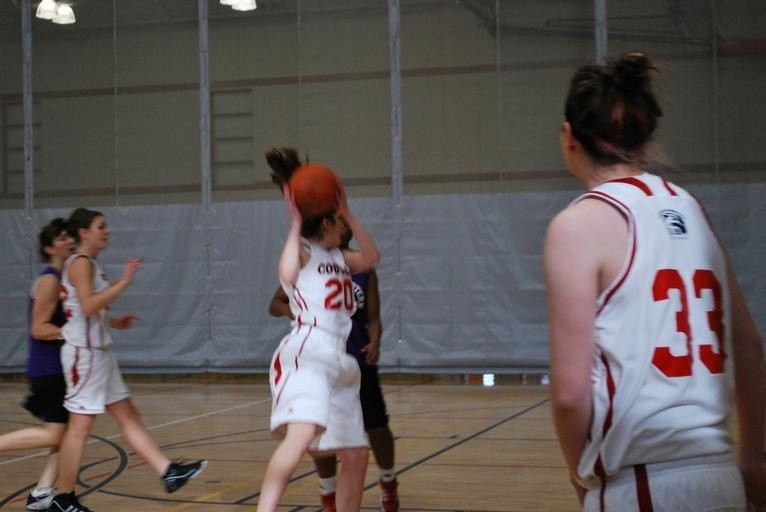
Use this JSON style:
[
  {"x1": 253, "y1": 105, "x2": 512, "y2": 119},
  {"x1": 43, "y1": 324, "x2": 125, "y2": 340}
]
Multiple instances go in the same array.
[{"x1": 289, "y1": 164, "x2": 339, "y2": 215}]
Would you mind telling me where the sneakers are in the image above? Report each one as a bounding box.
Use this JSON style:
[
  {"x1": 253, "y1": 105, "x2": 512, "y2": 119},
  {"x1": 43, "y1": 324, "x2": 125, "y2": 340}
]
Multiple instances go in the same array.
[
  {"x1": 49, "y1": 490, "x2": 91, "y2": 512},
  {"x1": 379, "y1": 477, "x2": 398, "y2": 512},
  {"x1": 26, "y1": 489, "x2": 55, "y2": 510},
  {"x1": 161, "y1": 459, "x2": 208, "y2": 492},
  {"x1": 320, "y1": 491, "x2": 336, "y2": 512}
]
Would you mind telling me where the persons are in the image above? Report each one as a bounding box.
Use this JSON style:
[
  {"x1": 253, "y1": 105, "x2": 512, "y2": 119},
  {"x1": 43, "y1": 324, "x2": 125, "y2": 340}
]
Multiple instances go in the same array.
[
  {"x1": 48, "y1": 207, "x2": 209, "y2": 512},
  {"x1": 252, "y1": 147, "x2": 385, "y2": 511},
  {"x1": 0, "y1": 217, "x2": 78, "y2": 511},
  {"x1": 540, "y1": 53, "x2": 763, "y2": 511},
  {"x1": 266, "y1": 214, "x2": 402, "y2": 510}
]
